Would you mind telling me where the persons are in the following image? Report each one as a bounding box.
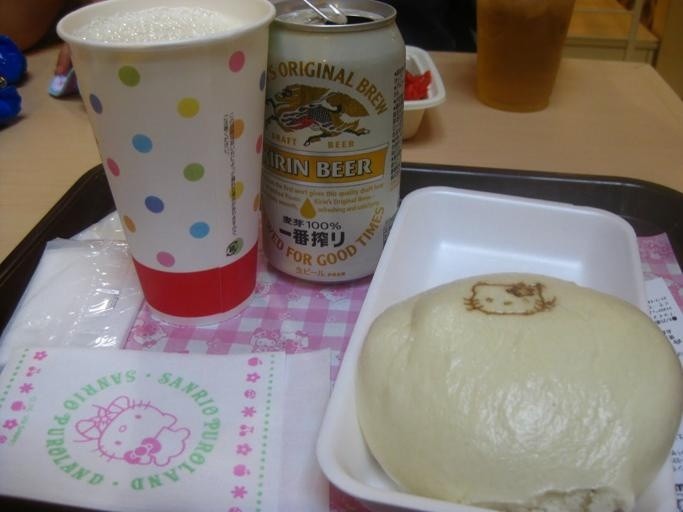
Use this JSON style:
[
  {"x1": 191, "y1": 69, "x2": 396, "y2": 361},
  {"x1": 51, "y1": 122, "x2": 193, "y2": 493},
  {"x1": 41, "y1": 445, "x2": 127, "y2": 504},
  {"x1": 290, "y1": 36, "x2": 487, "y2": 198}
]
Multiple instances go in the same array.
[{"x1": 0, "y1": 1, "x2": 92, "y2": 77}]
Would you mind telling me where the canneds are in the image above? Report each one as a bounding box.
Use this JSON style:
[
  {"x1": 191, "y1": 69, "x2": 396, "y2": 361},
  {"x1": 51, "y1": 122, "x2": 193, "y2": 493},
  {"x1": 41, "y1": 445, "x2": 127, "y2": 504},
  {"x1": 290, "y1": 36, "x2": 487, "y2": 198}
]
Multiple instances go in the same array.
[{"x1": 261, "y1": 0, "x2": 406, "y2": 285}]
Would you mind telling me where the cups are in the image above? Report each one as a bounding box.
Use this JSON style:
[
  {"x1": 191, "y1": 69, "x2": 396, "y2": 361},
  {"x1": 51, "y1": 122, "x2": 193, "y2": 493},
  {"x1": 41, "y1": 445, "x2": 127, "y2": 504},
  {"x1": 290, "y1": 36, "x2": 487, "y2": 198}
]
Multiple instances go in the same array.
[{"x1": 54, "y1": 0, "x2": 277, "y2": 326}]
